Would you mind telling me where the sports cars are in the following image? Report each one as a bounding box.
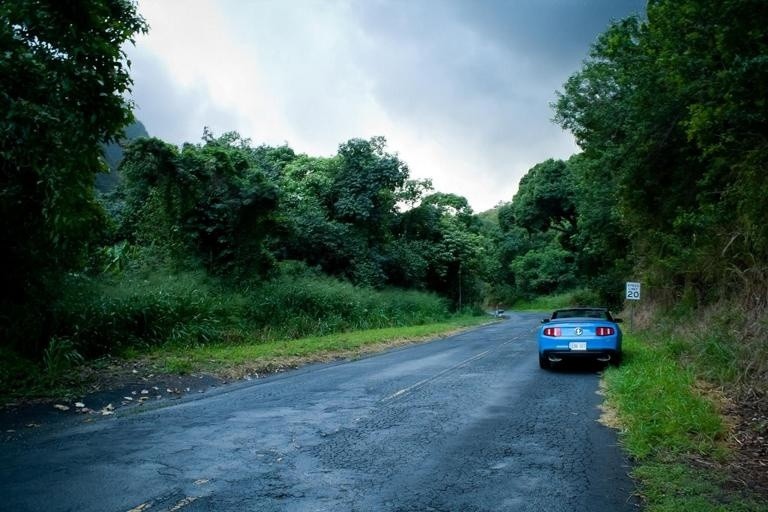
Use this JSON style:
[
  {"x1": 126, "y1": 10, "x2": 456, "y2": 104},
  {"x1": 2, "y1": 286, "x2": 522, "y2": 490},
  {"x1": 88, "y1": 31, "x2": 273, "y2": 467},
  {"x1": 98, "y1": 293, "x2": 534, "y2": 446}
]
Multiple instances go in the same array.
[{"x1": 538, "y1": 303, "x2": 625, "y2": 368}]
